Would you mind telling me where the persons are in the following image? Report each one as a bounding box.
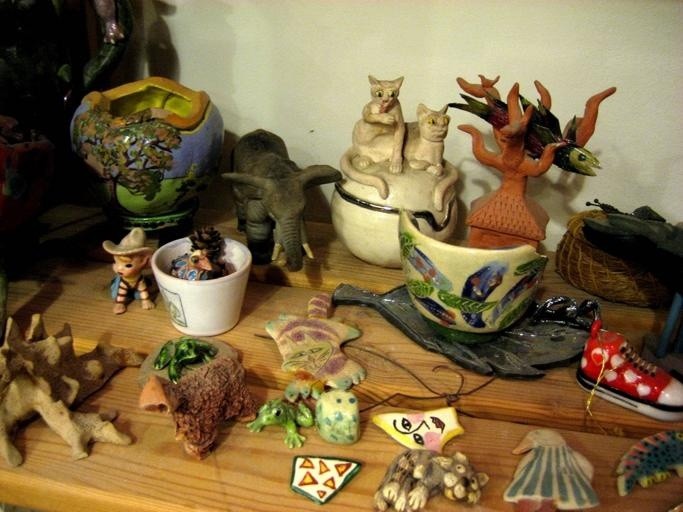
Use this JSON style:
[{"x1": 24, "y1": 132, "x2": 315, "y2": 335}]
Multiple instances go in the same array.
[{"x1": 102, "y1": 228, "x2": 155, "y2": 314}]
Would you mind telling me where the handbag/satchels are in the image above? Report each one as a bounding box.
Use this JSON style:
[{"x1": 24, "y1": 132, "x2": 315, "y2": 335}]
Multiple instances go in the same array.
[{"x1": 554, "y1": 209, "x2": 674, "y2": 308}]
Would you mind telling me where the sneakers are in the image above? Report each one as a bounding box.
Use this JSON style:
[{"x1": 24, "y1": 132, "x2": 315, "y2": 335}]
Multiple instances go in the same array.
[{"x1": 575, "y1": 319, "x2": 683, "y2": 423}]
